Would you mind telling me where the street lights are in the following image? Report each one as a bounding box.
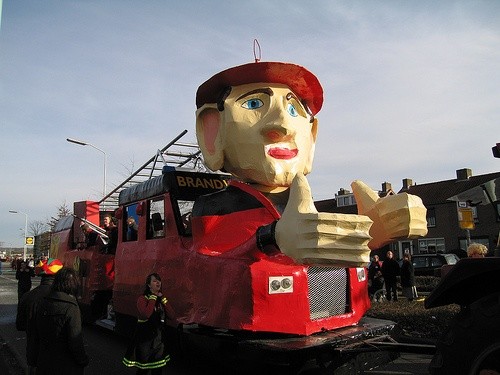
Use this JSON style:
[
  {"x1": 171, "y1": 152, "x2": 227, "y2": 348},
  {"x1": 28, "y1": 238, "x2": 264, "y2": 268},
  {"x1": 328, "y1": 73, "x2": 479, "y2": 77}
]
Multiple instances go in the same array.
[
  {"x1": 67, "y1": 138, "x2": 107, "y2": 211},
  {"x1": 9, "y1": 210, "x2": 27, "y2": 262}
]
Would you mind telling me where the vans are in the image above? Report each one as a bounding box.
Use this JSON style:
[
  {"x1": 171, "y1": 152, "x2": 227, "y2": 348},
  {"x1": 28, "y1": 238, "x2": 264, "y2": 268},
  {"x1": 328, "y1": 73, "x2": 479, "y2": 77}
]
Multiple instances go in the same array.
[{"x1": 397, "y1": 254, "x2": 460, "y2": 277}]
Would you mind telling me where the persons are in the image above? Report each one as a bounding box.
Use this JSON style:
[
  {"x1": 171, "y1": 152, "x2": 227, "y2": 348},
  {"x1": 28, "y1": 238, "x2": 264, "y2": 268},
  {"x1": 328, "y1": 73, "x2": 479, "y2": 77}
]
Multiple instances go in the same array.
[
  {"x1": 12, "y1": 257, "x2": 90, "y2": 375},
  {"x1": 122, "y1": 271, "x2": 173, "y2": 375},
  {"x1": 366, "y1": 250, "x2": 419, "y2": 300},
  {"x1": 466, "y1": 241, "x2": 488, "y2": 259},
  {"x1": 193, "y1": 38, "x2": 428, "y2": 272},
  {"x1": 79, "y1": 213, "x2": 119, "y2": 254}
]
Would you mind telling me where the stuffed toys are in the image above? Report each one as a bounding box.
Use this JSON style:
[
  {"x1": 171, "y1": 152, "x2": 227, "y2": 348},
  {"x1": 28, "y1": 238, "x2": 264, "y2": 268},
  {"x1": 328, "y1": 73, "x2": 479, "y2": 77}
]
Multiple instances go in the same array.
[{"x1": 126, "y1": 216, "x2": 139, "y2": 232}]
